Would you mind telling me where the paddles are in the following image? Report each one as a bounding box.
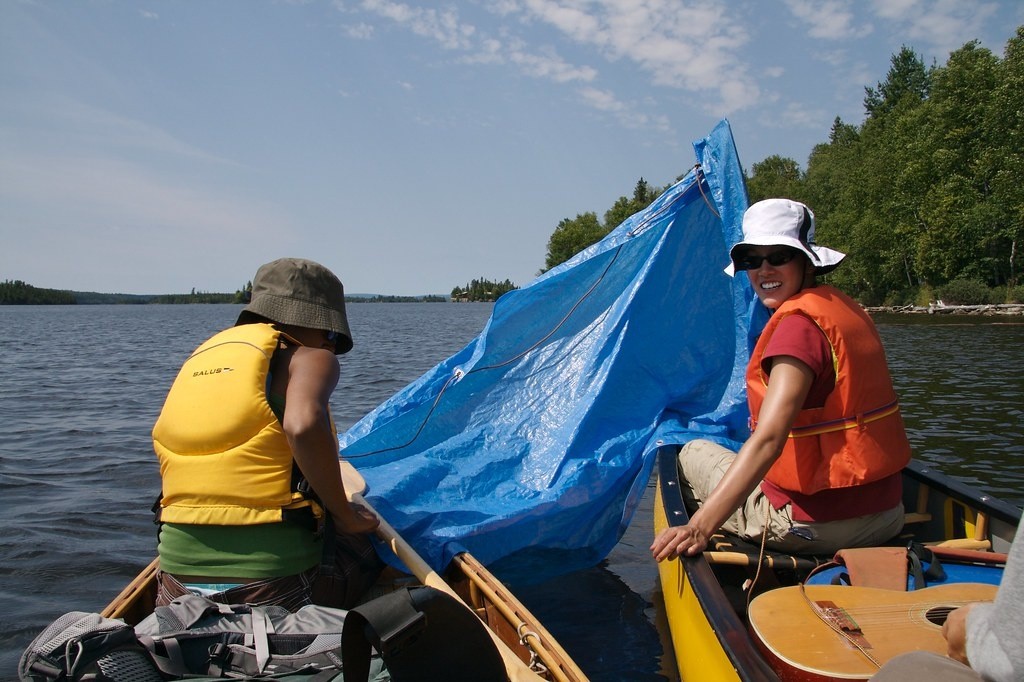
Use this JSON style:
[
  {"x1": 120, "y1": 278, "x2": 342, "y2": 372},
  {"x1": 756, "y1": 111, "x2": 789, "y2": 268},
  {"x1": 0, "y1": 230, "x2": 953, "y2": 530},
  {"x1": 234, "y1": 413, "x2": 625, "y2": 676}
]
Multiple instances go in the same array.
[{"x1": 336, "y1": 459, "x2": 552, "y2": 682}]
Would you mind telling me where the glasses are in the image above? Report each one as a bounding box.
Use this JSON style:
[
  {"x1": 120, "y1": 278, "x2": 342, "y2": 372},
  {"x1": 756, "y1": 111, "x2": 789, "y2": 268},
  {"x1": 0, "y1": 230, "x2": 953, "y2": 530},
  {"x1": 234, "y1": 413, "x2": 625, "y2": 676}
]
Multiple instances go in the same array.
[{"x1": 741, "y1": 247, "x2": 802, "y2": 270}]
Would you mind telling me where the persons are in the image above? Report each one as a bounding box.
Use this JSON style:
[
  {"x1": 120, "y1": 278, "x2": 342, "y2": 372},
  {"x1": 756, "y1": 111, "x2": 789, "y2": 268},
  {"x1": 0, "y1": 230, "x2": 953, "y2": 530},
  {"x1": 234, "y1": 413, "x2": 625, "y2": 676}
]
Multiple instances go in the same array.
[
  {"x1": 648, "y1": 197, "x2": 910, "y2": 565},
  {"x1": 154, "y1": 257, "x2": 386, "y2": 618},
  {"x1": 867, "y1": 501, "x2": 1024, "y2": 682}
]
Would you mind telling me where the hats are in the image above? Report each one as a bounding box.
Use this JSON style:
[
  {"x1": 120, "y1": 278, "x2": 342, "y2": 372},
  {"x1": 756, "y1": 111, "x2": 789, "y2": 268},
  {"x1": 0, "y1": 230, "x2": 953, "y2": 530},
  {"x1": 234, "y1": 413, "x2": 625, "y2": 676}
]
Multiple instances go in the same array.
[
  {"x1": 723, "y1": 198, "x2": 845, "y2": 279},
  {"x1": 233, "y1": 258, "x2": 355, "y2": 356}
]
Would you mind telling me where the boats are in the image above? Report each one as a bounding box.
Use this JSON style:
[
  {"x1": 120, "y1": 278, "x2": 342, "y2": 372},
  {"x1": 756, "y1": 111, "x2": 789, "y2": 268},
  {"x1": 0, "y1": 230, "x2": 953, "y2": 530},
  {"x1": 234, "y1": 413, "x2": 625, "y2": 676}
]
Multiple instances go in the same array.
[
  {"x1": 653, "y1": 443, "x2": 1024, "y2": 682},
  {"x1": 96, "y1": 554, "x2": 587, "y2": 682}
]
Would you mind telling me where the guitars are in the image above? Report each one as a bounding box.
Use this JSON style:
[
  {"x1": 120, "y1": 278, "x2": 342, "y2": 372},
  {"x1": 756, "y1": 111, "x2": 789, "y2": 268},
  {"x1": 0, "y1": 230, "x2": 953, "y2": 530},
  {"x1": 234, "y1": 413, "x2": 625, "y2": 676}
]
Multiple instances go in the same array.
[{"x1": 746, "y1": 582, "x2": 1001, "y2": 681}]
